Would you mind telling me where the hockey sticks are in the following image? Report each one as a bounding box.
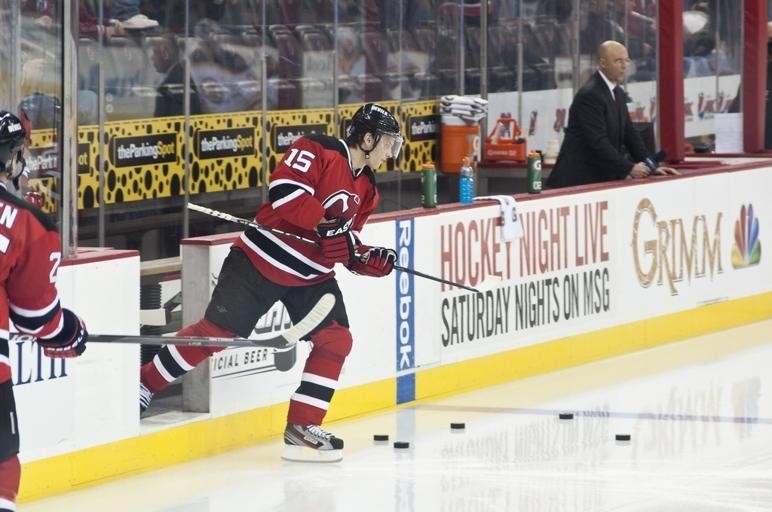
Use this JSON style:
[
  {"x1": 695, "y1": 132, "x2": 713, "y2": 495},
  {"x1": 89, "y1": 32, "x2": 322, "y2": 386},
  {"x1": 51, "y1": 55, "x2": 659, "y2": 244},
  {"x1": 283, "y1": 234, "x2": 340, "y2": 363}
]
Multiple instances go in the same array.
[
  {"x1": 8, "y1": 292, "x2": 336, "y2": 347},
  {"x1": 187, "y1": 202, "x2": 502, "y2": 293}
]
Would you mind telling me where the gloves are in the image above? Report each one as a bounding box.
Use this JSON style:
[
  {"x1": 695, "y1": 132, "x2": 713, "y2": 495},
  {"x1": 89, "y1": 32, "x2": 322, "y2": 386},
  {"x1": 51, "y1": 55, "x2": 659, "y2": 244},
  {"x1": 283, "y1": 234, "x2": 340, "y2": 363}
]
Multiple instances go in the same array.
[
  {"x1": 343, "y1": 238, "x2": 396, "y2": 277},
  {"x1": 39, "y1": 308, "x2": 87, "y2": 357}
]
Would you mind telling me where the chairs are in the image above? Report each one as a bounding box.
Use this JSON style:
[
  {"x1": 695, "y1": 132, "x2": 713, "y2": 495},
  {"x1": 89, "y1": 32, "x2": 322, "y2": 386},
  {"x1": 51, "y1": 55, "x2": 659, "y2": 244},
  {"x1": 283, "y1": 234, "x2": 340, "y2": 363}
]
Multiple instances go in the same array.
[{"x1": 41, "y1": 18, "x2": 560, "y2": 123}]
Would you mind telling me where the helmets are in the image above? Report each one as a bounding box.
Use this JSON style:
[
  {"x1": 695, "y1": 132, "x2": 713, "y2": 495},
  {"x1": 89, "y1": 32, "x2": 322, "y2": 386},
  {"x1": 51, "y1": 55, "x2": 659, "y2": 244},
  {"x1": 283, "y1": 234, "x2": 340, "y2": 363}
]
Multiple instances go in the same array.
[
  {"x1": 194, "y1": 18, "x2": 222, "y2": 53},
  {"x1": 0, "y1": 109, "x2": 25, "y2": 167},
  {"x1": 350, "y1": 103, "x2": 403, "y2": 160},
  {"x1": 333, "y1": 25, "x2": 359, "y2": 58}
]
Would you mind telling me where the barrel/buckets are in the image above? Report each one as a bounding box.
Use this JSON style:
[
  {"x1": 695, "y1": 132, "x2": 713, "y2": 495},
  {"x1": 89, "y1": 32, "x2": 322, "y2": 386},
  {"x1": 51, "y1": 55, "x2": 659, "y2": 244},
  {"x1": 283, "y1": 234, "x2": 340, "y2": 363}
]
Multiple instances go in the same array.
[{"x1": 439, "y1": 114, "x2": 480, "y2": 173}]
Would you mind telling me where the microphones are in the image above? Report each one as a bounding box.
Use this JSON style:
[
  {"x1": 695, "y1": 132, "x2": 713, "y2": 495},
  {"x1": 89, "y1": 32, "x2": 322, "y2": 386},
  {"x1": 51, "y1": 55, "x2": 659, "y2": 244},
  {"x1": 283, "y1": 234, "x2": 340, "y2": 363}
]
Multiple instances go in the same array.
[{"x1": 644, "y1": 150, "x2": 667, "y2": 170}]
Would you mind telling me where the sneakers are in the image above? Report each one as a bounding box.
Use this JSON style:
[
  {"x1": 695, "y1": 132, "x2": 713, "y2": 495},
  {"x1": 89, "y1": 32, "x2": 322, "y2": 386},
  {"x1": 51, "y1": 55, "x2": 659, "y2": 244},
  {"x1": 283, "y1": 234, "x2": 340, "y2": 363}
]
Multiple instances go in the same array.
[
  {"x1": 284, "y1": 421, "x2": 343, "y2": 450},
  {"x1": 141, "y1": 379, "x2": 153, "y2": 414},
  {"x1": 121, "y1": 14, "x2": 159, "y2": 29}
]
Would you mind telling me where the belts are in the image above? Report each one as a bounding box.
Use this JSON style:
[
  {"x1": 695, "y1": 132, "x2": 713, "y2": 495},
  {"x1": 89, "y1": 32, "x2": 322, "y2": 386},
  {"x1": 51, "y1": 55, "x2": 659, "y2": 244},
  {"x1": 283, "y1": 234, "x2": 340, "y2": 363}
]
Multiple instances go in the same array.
[{"x1": 317, "y1": 215, "x2": 356, "y2": 266}]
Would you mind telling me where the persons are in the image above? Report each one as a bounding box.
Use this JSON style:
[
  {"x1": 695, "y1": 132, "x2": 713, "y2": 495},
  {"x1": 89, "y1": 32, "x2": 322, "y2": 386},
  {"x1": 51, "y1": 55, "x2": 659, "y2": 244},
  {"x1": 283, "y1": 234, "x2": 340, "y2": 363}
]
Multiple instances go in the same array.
[
  {"x1": 0, "y1": 109, "x2": 89, "y2": 512},
  {"x1": 544, "y1": 38, "x2": 681, "y2": 189},
  {"x1": 138, "y1": 100, "x2": 399, "y2": 453}
]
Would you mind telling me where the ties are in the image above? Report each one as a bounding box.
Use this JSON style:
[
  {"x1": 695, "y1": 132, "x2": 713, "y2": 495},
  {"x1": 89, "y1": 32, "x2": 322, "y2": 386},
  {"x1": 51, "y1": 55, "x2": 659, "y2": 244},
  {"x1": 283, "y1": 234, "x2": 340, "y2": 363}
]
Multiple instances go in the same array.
[{"x1": 613, "y1": 86, "x2": 623, "y2": 112}]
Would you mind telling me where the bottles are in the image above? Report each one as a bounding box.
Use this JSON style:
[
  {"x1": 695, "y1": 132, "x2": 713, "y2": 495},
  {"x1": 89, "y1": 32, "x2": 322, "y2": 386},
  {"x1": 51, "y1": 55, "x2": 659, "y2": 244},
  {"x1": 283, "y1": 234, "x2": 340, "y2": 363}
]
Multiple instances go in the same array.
[
  {"x1": 458, "y1": 155, "x2": 475, "y2": 203},
  {"x1": 526, "y1": 149, "x2": 544, "y2": 195},
  {"x1": 419, "y1": 162, "x2": 438, "y2": 209}
]
